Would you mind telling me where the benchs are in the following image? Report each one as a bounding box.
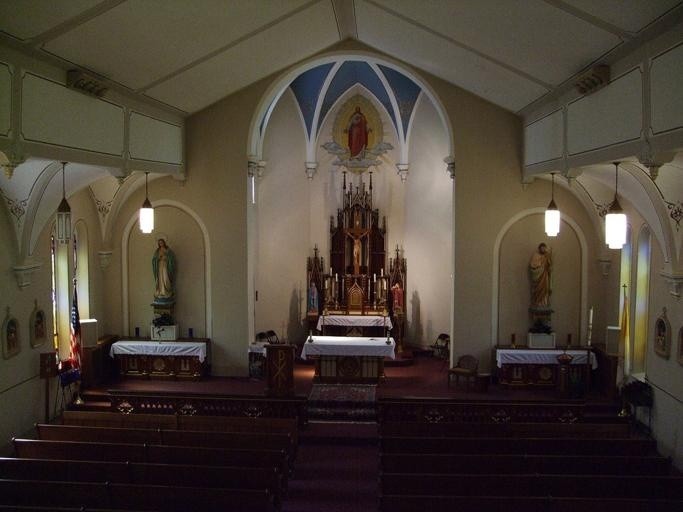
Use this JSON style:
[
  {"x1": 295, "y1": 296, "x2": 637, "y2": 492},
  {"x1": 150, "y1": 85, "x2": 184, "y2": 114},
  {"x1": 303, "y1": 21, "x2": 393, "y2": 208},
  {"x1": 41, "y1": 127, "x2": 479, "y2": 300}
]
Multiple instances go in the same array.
[
  {"x1": 0, "y1": 410, "x2": 299, "y2": 512},
  {"x1": 378, "y1": 421, "x2": 683, "y2": 512}
]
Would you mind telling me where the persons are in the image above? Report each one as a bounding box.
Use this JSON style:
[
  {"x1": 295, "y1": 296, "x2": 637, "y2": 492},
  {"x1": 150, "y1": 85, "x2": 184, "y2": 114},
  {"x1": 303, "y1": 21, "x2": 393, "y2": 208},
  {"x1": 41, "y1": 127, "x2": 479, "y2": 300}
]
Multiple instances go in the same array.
[
  {"x1": 343, "y1": 105, "x2": 374, "y2": 162},
  {"x1": 527, "y1": 241, "x2": 553, "y2": 310},
  {"x1": 342, "y1": 228, "x2": 371, "y2": 276},
  {"x1": 389, "y1": 282, "x2": 405, "y2": 317},
  {"x1": 307, "y1": 280, "x2": 319, "y2": 314},
  {"x1": 149, "y1": 238, "x2": 176, "y2": 301}
]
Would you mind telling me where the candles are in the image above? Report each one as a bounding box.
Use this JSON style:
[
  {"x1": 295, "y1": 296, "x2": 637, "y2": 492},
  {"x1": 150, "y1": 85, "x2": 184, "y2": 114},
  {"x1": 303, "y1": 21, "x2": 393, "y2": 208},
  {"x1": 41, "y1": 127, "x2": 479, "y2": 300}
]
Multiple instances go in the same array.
[
  {"x1": 324, "y1": 266, "x2": 340, "y2": 290},
  {"x1": 380, "y1": 267, "x2": 384, "y2": 277},
  {"x1": 589, "y1": 308, "x2": 593, "y2": 325},
  {"x1": 374, "y1": 273, "x2": 377, "y2": 283}
]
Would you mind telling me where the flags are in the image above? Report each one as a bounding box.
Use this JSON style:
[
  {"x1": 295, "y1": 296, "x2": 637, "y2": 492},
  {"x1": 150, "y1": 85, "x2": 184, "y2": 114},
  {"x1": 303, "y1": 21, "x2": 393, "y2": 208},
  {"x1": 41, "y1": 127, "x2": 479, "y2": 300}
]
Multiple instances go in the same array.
[{"x1": 67, "y1": 277, "x2": 86, "y2": 386}]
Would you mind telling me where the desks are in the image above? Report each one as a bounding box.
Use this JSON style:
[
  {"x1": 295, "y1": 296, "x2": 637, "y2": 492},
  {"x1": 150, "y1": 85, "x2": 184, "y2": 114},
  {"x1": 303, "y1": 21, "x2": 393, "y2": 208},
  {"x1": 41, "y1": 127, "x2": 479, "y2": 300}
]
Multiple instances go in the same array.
[
  {"x1": 495, "y1": 349, "x2": 598, "y2": 391},
  {"x1": 109, "y1": 341, "x2": 207, "y2": 382},
  {"x1": 249, "y1": 342, "x2": 270, "y2": 379},
  {"x1": 301, "y1": 315, "x2": 397, "y2": 381}
]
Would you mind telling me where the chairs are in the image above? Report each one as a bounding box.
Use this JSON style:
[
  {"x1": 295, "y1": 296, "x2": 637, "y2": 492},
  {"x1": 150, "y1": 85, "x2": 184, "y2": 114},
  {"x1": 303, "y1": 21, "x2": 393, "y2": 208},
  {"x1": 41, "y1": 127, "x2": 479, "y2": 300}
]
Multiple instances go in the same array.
[
  {"x1": 431, "y1": 333, "x2": 450, "y2": 371},
  {"x1": 257, "y1": 330, "x2": 281, "y2": 344},
  {"x1": 448, "y1": 355, "x2": 479, "y2": 393}
]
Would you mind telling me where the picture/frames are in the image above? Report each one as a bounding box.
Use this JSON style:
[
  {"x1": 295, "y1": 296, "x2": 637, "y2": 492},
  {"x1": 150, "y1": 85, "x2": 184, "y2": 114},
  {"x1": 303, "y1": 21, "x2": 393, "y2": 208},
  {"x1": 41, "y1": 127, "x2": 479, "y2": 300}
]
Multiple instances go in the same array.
[
  {"x1": 2, "y1": 315, "x2": 22, "y2": 359},
  {"x1": 29, "y1": 309, "x2": 47, "y2": 347},
  {"x1": 654, "y1": 314, "x2": 671, "y2": 359}
]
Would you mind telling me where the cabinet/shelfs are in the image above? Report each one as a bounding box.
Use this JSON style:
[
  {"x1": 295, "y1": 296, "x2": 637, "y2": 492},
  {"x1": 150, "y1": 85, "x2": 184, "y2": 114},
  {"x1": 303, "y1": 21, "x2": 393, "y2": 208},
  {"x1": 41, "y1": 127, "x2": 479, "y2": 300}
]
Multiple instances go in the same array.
[{"x1": 596, "y1": 342, "x2": 616, "y2": 368}]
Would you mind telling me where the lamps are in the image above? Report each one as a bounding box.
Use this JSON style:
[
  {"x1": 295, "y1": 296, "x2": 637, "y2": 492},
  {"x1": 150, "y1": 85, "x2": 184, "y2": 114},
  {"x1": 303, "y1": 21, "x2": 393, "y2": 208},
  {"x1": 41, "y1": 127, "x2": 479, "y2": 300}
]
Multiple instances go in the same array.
[
  {"x1": 544, "y1": 171, "x2": 561, "y2": 238},
  {"x1": 139, "y1": 170, "x2": 154, "y2": 234},
  {"x1": 55, "y1": 162, "x2": 72, "y2": 244},
  {"x1": 604, "y1": 161, "x2": 628, "y2": 250}
]
